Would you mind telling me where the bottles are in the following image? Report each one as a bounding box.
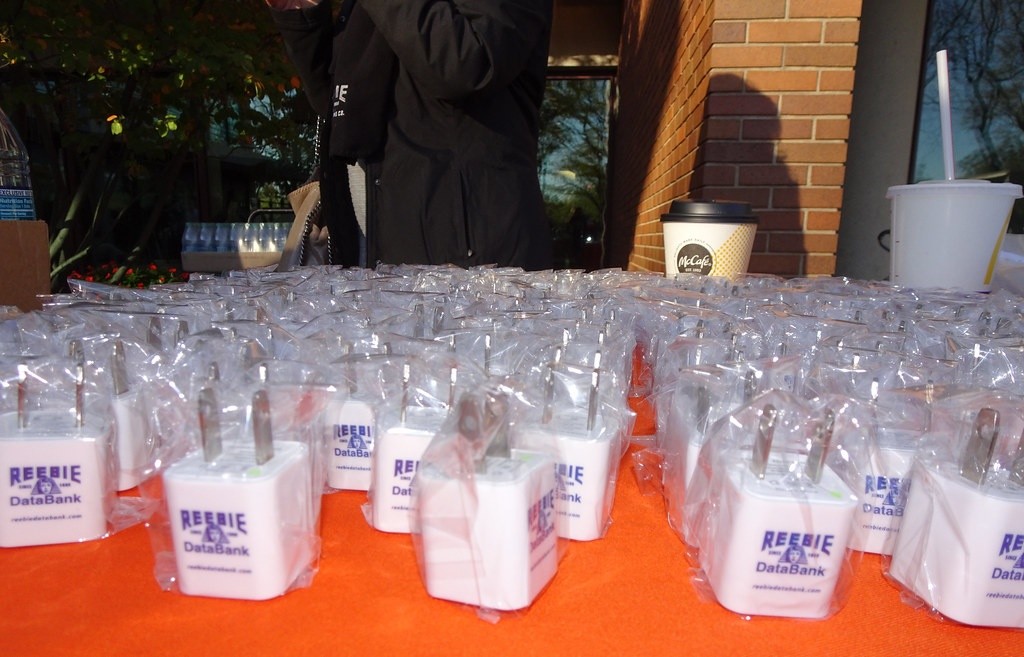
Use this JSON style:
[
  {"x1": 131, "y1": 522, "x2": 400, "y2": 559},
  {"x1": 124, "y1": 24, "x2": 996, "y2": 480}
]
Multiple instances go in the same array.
[
  {"x1": 182, "y1": 223, "x2": 293, "y2": 252},
  {"x1": 0, "y1": 107, "x2": 35, "y2": 221}
]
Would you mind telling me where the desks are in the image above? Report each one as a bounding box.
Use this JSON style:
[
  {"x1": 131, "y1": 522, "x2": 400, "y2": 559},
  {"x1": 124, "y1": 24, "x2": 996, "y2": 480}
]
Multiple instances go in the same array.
[{"x1": 0, "y1": 337, "x2": 1024, "y2": 657}]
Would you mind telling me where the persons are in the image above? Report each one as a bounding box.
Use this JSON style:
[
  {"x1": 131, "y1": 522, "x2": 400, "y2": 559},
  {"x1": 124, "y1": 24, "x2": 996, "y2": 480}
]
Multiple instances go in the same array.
[{"x1": 263, "y1": 0, "x2": 550, "y2": 267}]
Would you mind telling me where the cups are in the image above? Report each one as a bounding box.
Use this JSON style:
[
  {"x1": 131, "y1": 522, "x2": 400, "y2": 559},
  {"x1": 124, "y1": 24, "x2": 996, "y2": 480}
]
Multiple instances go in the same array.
[
  {"x1": 661, "y1": 200, "x2": 758, "y2": 281},
  {"x1": 887, "y1": 183, "x2": 1023, "y2": 293}
]
